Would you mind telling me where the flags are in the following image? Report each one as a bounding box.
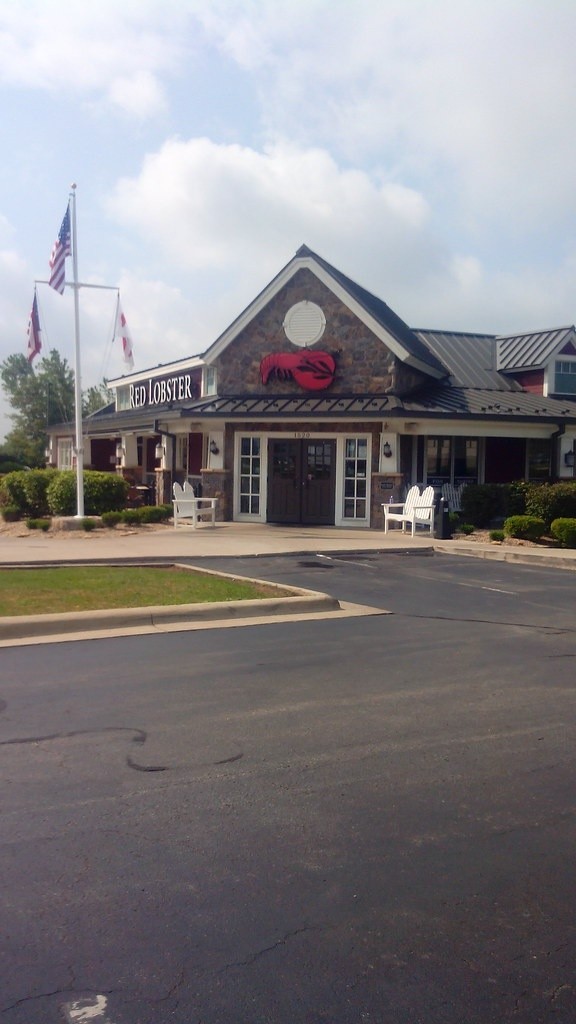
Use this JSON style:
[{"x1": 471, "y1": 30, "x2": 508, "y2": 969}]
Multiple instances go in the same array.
[
  {"x1": 113, "y1": 290, "x2": 134, "y2": 371},
  {"x1": 48, "y1": 199, "x2": 72, "y2": 294},
  {"x1": 26, "y1": 284, "x2": 42, "y2": 364}
]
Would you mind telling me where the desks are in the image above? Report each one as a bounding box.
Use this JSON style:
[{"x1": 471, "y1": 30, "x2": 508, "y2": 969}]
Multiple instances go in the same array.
[{"x1": 172, "y1": 481, "x2": 219, "y2": 529}]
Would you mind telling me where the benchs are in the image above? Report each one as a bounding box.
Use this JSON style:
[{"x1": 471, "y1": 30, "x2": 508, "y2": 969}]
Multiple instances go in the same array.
[
  {"x1": 442, "y1": 482, "x2": 468, "y2": 513},
  {"x1": 381, "y1": 485, "x2": 436, "y2": 537}
]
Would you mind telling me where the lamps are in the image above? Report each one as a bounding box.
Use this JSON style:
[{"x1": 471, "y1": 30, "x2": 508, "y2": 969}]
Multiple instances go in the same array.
[
  {"x1": 116, "y1": 445, "x2": 124, "y2": 459},
  {"x1": 45, "y1": 446, "x2": 53, "y2": 457},
  {"x1": 210, "y1": 439, "x2": 218, "y2": 453},
  {"x1": 155, "y1": 442, "x2": 165, "y2": 459},
  {"x1": 564, "y1": 450, "x2": 575, "y2": 466},
  {"x1": 384, "y1": 442, "x2": 392, "y2": 458}
]
